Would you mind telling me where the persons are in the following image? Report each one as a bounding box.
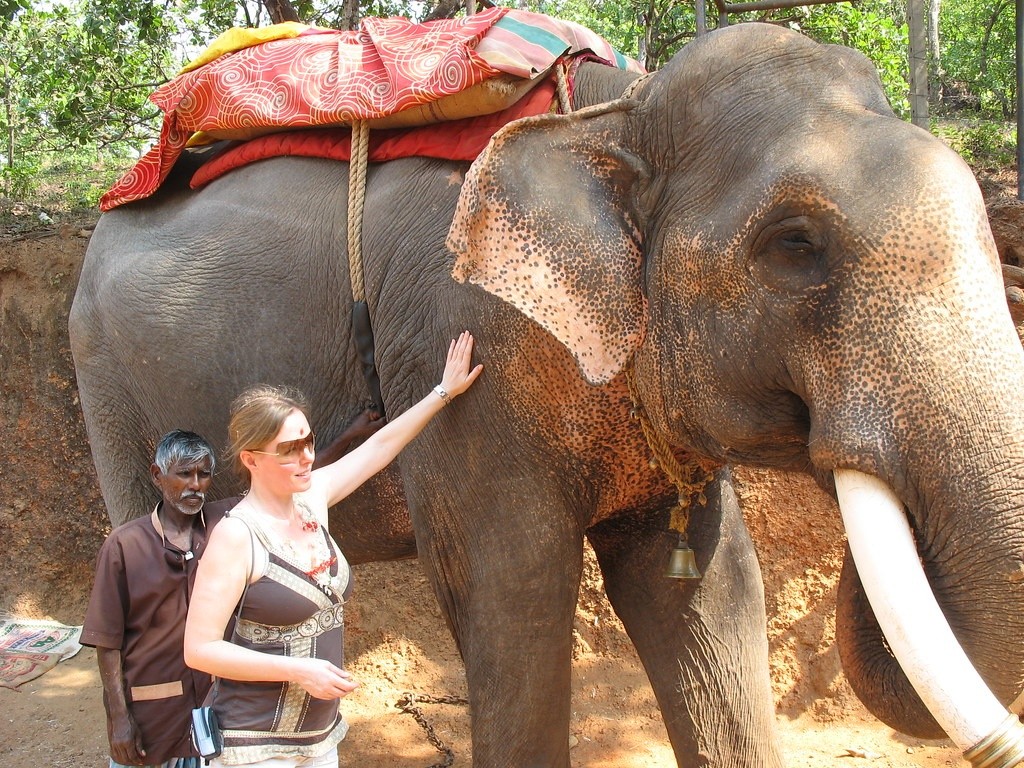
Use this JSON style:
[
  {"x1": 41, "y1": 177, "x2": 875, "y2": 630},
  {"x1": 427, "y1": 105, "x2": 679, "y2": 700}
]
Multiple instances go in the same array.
[
  {"x1": 79, "y1": 404, "x2": 387, "y2": 768},
  {"x1": 185, "y1": 330, "x2": 484, "y2": 768}
]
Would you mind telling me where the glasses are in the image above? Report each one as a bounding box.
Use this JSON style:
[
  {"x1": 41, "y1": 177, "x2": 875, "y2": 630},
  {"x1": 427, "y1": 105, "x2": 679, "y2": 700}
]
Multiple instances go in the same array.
[{"x1": 244, "y1": 431, "x2": 315, "y2": 461}]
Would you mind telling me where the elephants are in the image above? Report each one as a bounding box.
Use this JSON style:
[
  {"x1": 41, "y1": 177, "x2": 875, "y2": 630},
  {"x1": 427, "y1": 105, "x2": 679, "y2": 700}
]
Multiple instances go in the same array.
[{"x1": 69, "y1": 22, "x2": 1024, "y2": 767}]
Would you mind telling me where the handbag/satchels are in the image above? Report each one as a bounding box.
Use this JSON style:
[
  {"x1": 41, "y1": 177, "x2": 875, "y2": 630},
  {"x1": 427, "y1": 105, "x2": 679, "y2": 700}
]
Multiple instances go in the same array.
[{"x1": 189, "y1": 706, "x2": 222, "y2": 760}]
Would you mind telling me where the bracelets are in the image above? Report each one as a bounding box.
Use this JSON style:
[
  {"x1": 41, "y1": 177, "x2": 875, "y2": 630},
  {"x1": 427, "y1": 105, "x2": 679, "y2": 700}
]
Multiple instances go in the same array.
[{"x1": 433, "y1": 385, "x2": 450, "y2": 405}]
